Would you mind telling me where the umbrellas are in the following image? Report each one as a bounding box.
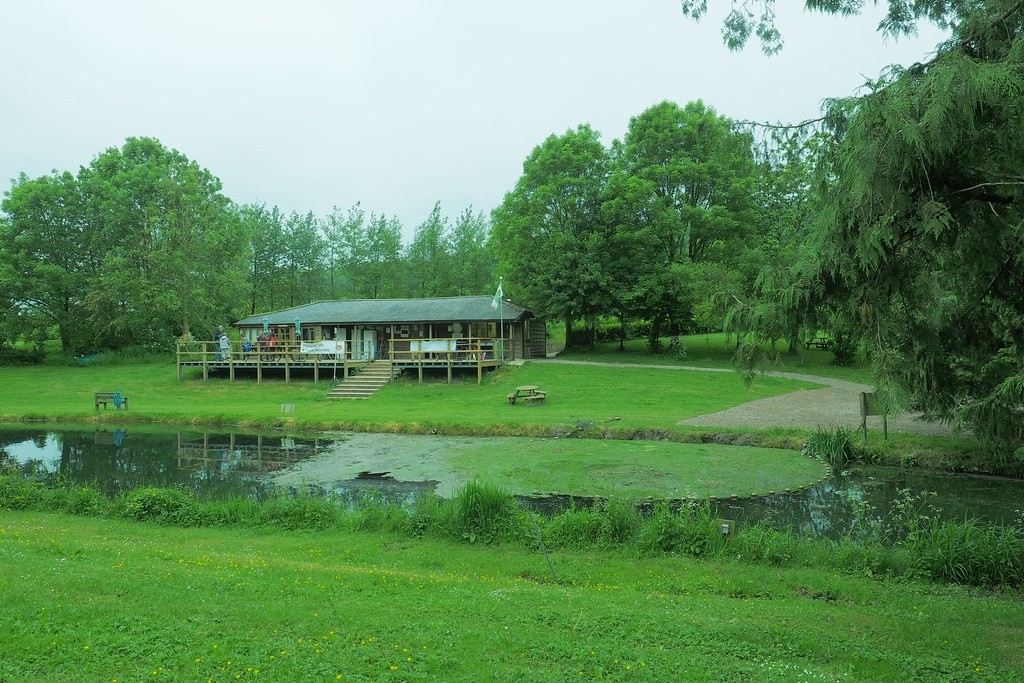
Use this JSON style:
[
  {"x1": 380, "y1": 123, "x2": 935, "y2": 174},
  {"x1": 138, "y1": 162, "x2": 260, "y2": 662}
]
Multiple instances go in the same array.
[
  {"x1": 294, "y1": 314, "x2": 304, "y2": 362},
  {"x1": 262, "y1": 315, "x2": 270, "y2": 336}
]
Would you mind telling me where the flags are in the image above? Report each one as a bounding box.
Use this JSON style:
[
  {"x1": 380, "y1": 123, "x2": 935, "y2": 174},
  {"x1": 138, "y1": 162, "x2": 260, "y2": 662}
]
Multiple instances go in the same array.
[{"x1": 491, "y1": 281, "x2": 504, "y2": 311}]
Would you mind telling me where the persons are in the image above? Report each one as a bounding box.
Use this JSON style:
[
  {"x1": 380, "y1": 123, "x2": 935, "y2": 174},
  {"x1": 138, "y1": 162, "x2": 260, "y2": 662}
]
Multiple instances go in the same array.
[
  {"x1": 213, "y1": 325, "x2": 232, "y2": 362},
  {"x1": 239, "y1": 328, "x2": 278, "y2": 362}
]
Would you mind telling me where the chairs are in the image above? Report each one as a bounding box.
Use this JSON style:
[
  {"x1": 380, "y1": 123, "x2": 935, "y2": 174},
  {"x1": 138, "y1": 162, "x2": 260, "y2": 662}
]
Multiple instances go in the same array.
[
  {"x1": 250, "y1": 346, "x2": 297, "y2": 362},
  {"x1": 438, "y1": 346, "x2": 483, "y2": 361}
]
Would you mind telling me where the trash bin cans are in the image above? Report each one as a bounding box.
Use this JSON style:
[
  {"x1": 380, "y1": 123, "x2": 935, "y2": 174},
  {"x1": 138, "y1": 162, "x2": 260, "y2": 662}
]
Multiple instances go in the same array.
[{"x1": 491, "y1": 339, "x2": 507, "y2": 360}]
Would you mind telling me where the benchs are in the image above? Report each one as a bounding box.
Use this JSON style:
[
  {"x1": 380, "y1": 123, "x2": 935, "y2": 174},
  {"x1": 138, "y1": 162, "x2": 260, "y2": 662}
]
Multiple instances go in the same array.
[
  {"x1": 507, "y1": 390, "x2": 546, "y2": 405},
  {"x1": 806, "y1": 341, "x2": 828, "y2": 347},
  {"x1": 94, "y1": 392, "x2": 128, "y2": 411}
]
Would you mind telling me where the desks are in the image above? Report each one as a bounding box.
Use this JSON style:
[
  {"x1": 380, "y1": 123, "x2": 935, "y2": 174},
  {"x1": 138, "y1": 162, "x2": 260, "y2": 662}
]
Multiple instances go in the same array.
[
  {"x1": 806, "y1": 337, "x2": 828, "y2": 350},
  {"x1": 511, "y1": 386, "x2": 539, "y2": 403}
]
